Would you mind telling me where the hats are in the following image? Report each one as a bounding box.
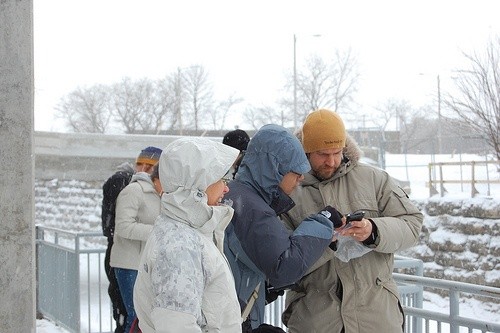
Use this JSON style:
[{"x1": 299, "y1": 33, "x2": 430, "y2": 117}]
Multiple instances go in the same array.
[
  {"x1": 136, "y1": 146, "x2": 163, "y2": 165},
  {"x1": 301, "y1": 109, "x2": 347, "y2": 153},
  {"x1": 151, "y1": 163, "x2": 159, "y2": 178},
  {"x1": 223, "y1": 128, "x2": 250, "y2": 153}
]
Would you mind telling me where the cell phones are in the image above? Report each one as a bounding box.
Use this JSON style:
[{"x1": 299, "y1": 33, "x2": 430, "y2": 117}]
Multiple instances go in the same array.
[{"x1": 346, "y1": 211, "x2": 365, "y2": 225}]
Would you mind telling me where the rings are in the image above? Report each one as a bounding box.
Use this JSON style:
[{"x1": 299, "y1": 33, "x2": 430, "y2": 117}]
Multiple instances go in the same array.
[
  {"x1": 363, "y1": 219, "x2": 367, "y2": 227},
  {"x1": 352, "y1": 231, "x2": 356, "y2": 238}
]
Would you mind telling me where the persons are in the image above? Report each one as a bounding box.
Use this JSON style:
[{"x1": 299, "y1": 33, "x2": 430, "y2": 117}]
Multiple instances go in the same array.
[
  {"x1": 221, "y1": 128, "x2": 251, "y2": 178},
  {"x1": 283, "y1": 110, "x2": 425, "y2": 333},
  {"x1": 101, "y1": 145, "x2": 162, "y2": 333},
  {"x1": 219, "y1": 124, "x2": 346, "y2": 333},
  {"x1": 132, "y1": 137, "x2": 242, "y2": 333},
  {"x1": 109, "y1": 163, "x2": 162, "y2": 333}
]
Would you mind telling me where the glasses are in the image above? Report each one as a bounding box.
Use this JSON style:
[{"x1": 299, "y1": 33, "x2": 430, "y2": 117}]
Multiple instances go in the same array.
[{"x1": 221, "y1": 177, "x2": 230, "y2": 185}]
